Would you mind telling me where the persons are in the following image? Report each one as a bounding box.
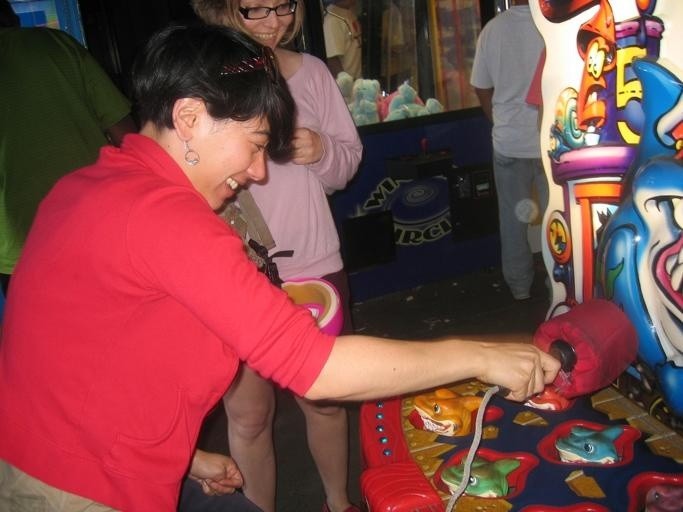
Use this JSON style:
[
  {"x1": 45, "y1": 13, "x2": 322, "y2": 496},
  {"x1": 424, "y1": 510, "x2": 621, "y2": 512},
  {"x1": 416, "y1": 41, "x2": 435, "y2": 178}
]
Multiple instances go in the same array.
[
  {"x1": 323, "y1": 0, "x2": 364, "y2": 80},
  {"x1": 0, "y1": 0, "x2": 138, "y2": 302},
  {"x1": 0, "y1": 18, "x2": 564, "y2": 511},
  {"x1": 196, "y1": 1, "x2": 364, "y2": 511},
  {"x1": 470, "y1": 1, "x2": 547, "y2": 302}
]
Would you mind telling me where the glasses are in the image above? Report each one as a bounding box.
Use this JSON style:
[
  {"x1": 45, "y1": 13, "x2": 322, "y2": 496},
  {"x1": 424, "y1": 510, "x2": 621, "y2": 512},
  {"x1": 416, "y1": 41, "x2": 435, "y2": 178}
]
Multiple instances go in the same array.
[{"x1": 237, "y1": 2, "x2": 295, "y2": 19}]
